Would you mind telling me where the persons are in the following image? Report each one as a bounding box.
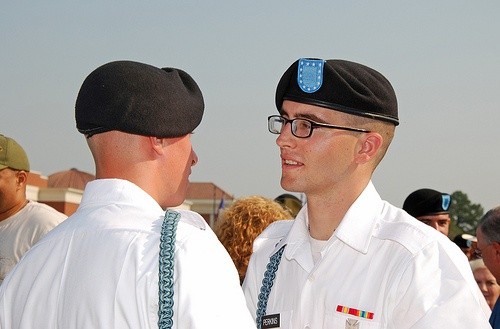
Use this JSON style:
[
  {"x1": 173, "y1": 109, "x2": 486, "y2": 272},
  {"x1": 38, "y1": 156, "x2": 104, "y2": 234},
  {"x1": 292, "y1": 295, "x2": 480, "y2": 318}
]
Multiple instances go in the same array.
[
  {"x1": 402, "y1": 189, "x2": 451, "y2": 241},
  {"x1": 476, "y1": 207, "x2": 500, "y2": 329},
  {"x1": 452, "y1": 234, "x2": 478, "y2": 262},
  {"x1": 240, "y1": 58, "x2": 493, "y2": 329},
  {"x1": 0, "y1": 61, "x2": 255, "y2": 329},
  {"x1": 0, "y1": 134, "x2": 70, "y2": 285},
  {"x1": 468, "y1": 257, "x2": 499, "y2": 309},
  {"x1": 216, "y1": 195, "x2": 293, "y2": 286}
]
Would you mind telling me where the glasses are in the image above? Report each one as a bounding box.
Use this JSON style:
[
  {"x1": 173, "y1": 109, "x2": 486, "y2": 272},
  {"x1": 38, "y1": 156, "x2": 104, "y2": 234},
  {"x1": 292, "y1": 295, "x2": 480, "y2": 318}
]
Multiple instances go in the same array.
[{"x1": 267, "y1": 115, "x2": 371, "y2": 139}]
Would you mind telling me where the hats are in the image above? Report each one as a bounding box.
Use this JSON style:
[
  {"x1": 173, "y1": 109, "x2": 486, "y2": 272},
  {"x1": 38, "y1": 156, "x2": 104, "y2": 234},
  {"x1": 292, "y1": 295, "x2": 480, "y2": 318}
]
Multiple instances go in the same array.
[
  {"x1": 0, "y1": 134, "x2": 30, "y2": 174},
  {"x1": 452, "y1": 233, "x2": 475, "y2": 248},
  {"x1": 403, "y1": 188, "x2": 451, "y2": 217},
  {"x1": 275, "y1": 57, "x2": 400, "y2": 125},
  {"x1": 75, "y1": 61, "x2": 205, "y2": 138}
]
